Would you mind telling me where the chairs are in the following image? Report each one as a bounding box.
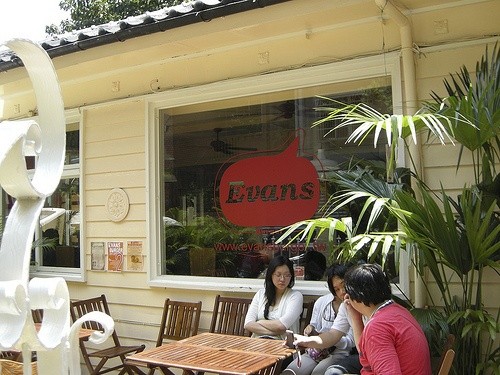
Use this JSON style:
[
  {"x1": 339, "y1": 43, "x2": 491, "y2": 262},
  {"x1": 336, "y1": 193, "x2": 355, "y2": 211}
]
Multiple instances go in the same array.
[
  {"x1": 69, "y1": 294, "x2": 146, "y2": 375},
  {"x1": 299, "y1": 299, "x2": 315, "y2": 336},
  {"x1": 147, "y1": 298, "x2": 202, "y2": 375},
  {"x1": 433, "y1": 333, "x2": 455, "y2": 375},
  {"x1": 209, "y1": 295, "x2": 253, "y2": 338}
]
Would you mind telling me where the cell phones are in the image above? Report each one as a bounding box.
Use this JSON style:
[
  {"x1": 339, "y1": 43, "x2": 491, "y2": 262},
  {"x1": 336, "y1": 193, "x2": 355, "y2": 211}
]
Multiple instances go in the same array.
[{"x1": 286, "y1": 330, "x2": 295, "y2": 349}]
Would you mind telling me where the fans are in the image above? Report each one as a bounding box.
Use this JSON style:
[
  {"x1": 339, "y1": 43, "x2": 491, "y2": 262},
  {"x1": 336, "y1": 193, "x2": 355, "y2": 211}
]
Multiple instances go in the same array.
[
  {"x1": 186, "y1": 127, "x2": 259, "y2": 157},
  {"x1": 232, "y1": 99, "x2": 329, "y2": 126}
]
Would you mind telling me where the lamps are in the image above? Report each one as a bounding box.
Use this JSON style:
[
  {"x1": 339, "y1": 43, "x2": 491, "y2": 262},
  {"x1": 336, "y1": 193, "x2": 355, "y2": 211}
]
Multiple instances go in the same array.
[{"x1": 306, "y1": 142, "x2": 340, "y2": 171}]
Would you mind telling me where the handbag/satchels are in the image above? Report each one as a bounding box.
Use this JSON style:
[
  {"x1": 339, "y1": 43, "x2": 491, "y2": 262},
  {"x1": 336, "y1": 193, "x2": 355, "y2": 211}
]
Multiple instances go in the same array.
[{"x1": 304, "y1": 333, "x2": 337, "y2": 363}]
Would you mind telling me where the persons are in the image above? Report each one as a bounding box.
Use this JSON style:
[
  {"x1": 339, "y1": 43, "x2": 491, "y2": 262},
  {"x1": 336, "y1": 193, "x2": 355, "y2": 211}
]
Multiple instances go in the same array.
[
  {"x1": 244, "y1": 256, "x2": 303, "y2": 348},
  {"x1": 344, "y1": 263, "x2": 432, "y2": 375},
  {"x1": 41, "y1": 228, "x2": 62, "y2": 266},
  {"x1": 281, "y1": 263, "x2": 369, "y2": 375}
]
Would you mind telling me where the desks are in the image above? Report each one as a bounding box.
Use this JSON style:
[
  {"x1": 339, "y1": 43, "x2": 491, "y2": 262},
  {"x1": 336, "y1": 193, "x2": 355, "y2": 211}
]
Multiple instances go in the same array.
[
  {"x1": 126, "y1": 332, "x2": 298, "y2": 375},
  {"x1": 33, "y1": 322, "x2": 104, "y2": 339}
]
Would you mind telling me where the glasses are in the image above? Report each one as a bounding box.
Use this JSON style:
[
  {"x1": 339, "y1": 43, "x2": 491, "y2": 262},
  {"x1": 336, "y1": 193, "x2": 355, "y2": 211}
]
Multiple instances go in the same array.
[{"x1": 272, "y1": 272, "x2": 293, "y2": 278}]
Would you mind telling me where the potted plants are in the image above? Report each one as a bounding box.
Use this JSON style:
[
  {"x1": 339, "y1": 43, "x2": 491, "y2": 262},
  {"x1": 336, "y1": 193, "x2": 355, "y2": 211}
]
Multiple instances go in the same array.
[{"x1": 161, "y1": 207, "x2": 259, "y2": 276}]
